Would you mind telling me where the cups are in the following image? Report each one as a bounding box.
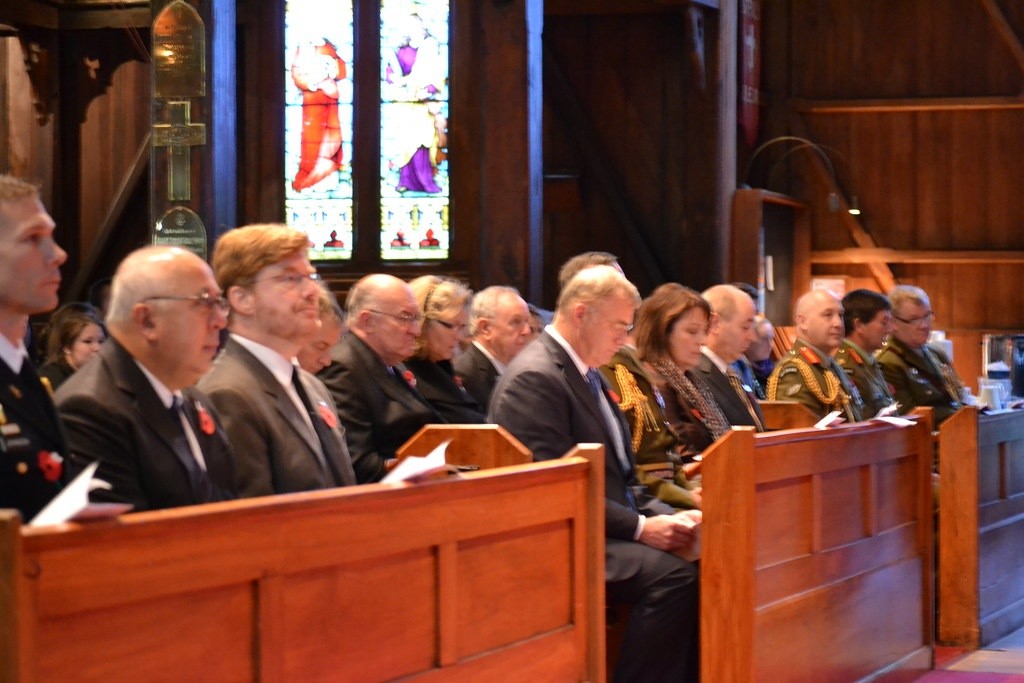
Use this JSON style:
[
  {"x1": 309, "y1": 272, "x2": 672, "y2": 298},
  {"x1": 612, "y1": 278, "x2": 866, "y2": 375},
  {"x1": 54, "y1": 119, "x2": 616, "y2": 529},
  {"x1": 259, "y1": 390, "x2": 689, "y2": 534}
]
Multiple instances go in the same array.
[{"x1": 980, "y1": 386, "x2": 1001, "y2": 410}]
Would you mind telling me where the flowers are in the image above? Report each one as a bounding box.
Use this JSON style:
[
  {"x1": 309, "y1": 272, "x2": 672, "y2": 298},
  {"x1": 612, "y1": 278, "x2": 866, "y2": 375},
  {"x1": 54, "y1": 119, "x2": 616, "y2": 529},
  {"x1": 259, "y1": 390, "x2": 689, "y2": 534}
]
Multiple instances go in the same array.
[
  {"x1": 402, "y1": 370, "x2": 417, "y2": 387},
  {"x1": 39, "y1": 451, "x2": 63, "y2": 483},
  {"x1": 194, "y1": 402, "x2": 216, "y2": 434},
  {"x1": 320, "y1": 403, "x2": 337, "y2": 431},
  {"x1": 454, "y1": 375, "x2": 468, "y2": 393}
]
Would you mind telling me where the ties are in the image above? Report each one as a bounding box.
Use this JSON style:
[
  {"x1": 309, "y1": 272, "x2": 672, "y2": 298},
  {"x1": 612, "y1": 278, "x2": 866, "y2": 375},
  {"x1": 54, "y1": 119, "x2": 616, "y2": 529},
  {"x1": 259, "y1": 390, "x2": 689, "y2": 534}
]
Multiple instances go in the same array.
[
  {"x1": 727, "y1": 366, "x2": 765, "y2": 432},
  {"x1": 586, "y1": 370, "x2": 630, "y2": 486},
  {"x1": 289, "y1": 368, "x2": 347, "y2": 489}
]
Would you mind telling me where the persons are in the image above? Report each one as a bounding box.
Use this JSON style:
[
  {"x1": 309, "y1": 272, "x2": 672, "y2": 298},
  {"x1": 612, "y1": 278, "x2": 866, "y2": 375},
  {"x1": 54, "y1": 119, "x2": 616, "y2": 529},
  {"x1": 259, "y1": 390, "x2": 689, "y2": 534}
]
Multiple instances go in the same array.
[
  {"x1": 43, "y1": 222, "x2": 964, "y2": 683},
  {"x1": 1, "y1": 173, "x2": 79, "y2": 522}
]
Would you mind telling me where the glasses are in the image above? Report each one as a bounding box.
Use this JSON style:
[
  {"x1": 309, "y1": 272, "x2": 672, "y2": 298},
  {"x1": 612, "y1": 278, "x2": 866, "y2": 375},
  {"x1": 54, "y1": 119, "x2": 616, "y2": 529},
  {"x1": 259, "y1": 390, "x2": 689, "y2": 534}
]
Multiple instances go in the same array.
[
  {"x1": 245, "y1": 271, "x2": 322, "y2": 287},
  {"x1": 891, "y1": 312, "x2": 936, "y2": 327},
  {"x1": 140, "y1": 294, "x2": 231, "y2": 318},
  {"x1": 433, "y1": 318, "x2": 469, "y2": 338},
  {"x1": 366, "y1": 308, "x2": 424, "y2": 327}
]
